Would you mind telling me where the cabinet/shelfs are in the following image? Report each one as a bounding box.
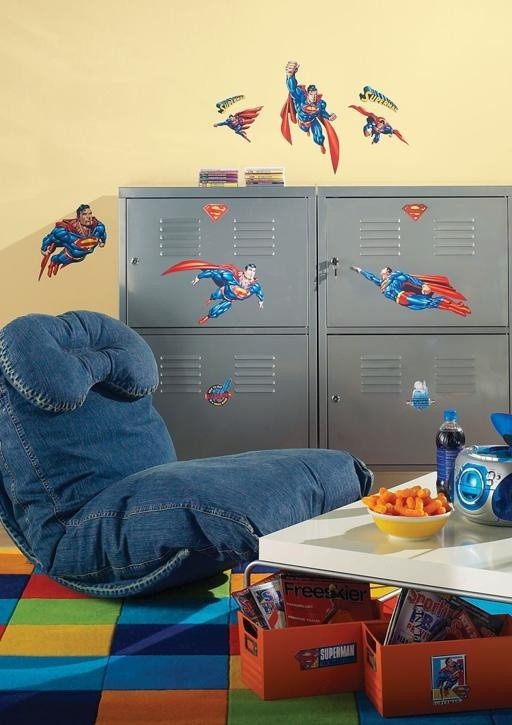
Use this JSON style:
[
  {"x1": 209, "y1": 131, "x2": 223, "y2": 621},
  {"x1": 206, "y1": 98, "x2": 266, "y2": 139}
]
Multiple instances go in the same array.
[
  {"x1": 117, "y1": 183, "x2": 318, "y2": 461},
  {"x1": 318, "y1": 186, "x2": 512, "y2": 471}
]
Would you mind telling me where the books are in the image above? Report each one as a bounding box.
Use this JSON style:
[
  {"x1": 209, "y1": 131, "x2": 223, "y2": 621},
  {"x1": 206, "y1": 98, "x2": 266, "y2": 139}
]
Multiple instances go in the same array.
[
  {"x1": 197, "y1": 161, "x2": 286, "y2": 187},
  {"x1": 230, "y1": 569, "x2": 505, "y2": 649}
]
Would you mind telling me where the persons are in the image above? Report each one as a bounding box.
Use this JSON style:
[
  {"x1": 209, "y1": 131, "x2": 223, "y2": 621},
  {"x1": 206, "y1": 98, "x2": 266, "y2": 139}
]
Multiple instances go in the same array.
[
  {"x1": 41, "y1": 203, "x2": 106, "y2": 277},
  {"x1": 436, "y1": 657, "x2": 464, "y2": 697},
  {"x1": 348, "y1": 265, "x2": 472, "y2": 317},
  {"x1": 362, "y1": 115, "x2": 394, "y2": 144},
  {"x1": 285, "y1": 59, "x2": 335, "y2": 157},
  {"x1": 212, "y1": 110, "x2": 251, "y2": 143},
  {"x1": 189, "y1": 260, "x2": 264, "y2": 326}
]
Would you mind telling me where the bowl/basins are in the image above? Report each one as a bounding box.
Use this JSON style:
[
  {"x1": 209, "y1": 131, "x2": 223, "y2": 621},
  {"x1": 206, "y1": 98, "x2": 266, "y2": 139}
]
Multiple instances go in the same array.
[{"x1": 366, "y1": 504, "x2": 453, "y2": 540}]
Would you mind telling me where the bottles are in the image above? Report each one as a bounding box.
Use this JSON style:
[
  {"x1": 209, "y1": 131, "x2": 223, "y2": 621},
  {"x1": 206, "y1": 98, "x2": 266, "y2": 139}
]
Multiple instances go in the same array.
[{"x1": 435, "y1": 410, "x2": 465, "y2": 503}]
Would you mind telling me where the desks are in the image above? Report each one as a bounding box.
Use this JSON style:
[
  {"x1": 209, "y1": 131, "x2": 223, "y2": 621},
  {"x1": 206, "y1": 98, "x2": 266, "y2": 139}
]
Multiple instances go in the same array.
[{"x1": 243, "y1": 469, "x2": 512, "y2": 607}]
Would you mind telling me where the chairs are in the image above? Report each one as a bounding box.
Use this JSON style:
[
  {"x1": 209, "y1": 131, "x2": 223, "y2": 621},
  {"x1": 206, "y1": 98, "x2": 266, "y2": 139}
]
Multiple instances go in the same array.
[{"x1": 0, "y1": 309, "x2": 376, "y2": 600}]
